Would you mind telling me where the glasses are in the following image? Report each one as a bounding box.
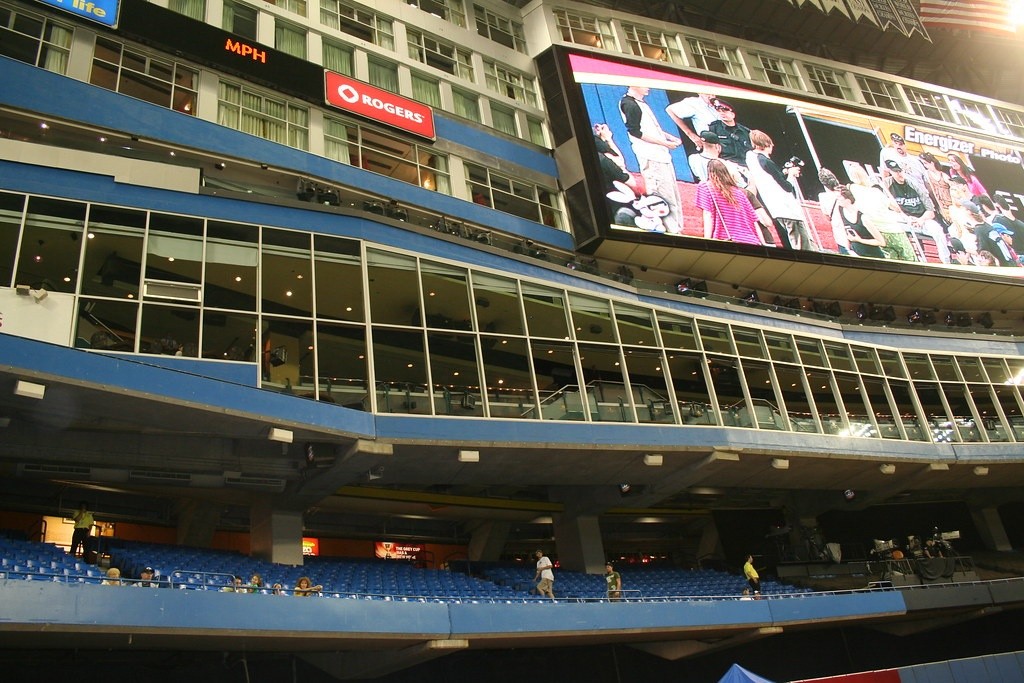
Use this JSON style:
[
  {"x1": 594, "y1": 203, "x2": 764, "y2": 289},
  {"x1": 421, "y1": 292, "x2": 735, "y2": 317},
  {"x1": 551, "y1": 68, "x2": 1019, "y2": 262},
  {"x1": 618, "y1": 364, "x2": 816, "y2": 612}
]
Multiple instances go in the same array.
[
  {"x1": 919, "y1": 153, "x2": 934, "y2": 163},
  {"x1": 715, "y1": 105, "x2": 729, "y2": 111}
]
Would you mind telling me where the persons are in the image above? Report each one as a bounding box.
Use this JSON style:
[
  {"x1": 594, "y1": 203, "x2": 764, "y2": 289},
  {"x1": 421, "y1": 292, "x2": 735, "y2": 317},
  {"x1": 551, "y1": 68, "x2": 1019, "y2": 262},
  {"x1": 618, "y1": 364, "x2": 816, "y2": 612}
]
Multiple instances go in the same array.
[
  {"x1": 740, "y1": 589, "x2": 754, "y2": 600},
  {"x1": 70, "y1": 501, "x2": 94, "y2": 558},
  {"x1": 605, "y1": 562, "x2": 622, "y2": 602},
  {"x1": 744, "y1": 554, "x2": 762, "y2": 600},
  {"x1": 533, "y1": 550, "x2": 555, "y2": 598},
  {"x1": 294, "y1": 577, "x2": 322, "y2": 597},
  {"x1": 924, "y1": 540, "x2": 945, "y2": 559},
  {"x1": 161, "y1": 331, "x2": 183, "y2": 355},
  {"x1": 101, "y1": 568, "x2": 125, "y2": 586},
  {"x1": 223, "y1": 574, "x2": 283, "y2": 595},
  {"x1": 591, "y1": 86, "x2": 1024, "y2": 268},
  {"x1": 132, "y1": 566, "x2": 155, "y2": 588}
]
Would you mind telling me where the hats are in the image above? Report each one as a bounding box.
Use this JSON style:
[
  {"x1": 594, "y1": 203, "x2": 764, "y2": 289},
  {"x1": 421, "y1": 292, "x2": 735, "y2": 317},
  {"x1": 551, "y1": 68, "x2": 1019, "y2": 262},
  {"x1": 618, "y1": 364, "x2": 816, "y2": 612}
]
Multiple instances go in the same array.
[
  {"x1": 890, "y1": 133, "x2": 904, "y2": 144},
  {"x1": 948, "y1": 177, "x2": 966, "y2": 185},
  {"x1": 992, "y1": 223, "x2": 1014, "y2": 235},
  {"x1": 141, "y1": 566, "x2": 155, "y2": 574},
  {"x1": 885, "y1": 160, "x2": 903, "y2": 172},
  {"x1": 700, "y1": 130, "x2": 726, "y2": 148},
  {"x1": 710, "y1": 98, "x2": 734, "y2": 109}
]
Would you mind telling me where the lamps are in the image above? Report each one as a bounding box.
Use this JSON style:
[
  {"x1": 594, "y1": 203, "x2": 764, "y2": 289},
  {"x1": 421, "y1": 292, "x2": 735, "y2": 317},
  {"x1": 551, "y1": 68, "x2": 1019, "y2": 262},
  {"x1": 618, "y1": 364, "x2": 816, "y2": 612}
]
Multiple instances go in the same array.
[
  {"x1": 457, "y1": 450, "x2": 479, "y2": 462},
  {"x1": 642, "y1": 265, "x2": 649, "y2": 271},
  {"x1": 261, "y1": 163, "x2": 269, "y2": 171},
  {"x1": 527, "y1": 238, "x2": 534, "y2": 247},
  {"x1": 982, "y1": 418, "x2": 996, "y2": 431},
  {"x1": 461, "y1": 390, "x2": 476, "y2": 410},
  {"x1": 733, "y1": 283, "x2": 740, "y2": 289},
  {"x1": 644, "y1": 453, "x2": 663, "y2": 465},
  {"x1": 34, "y1": 288, "x2": 48, "y2": 304},
  {"x1": 972, "y1": 465, "x2": 989, "y2": 475},
  {"x1": 390, "y1": 198, "x2": 399, "y2": 205},
  {"x1": 16, "y1": 284, "x2": 30, "y2": 296},
  {"x1": 12, "y1": 380, "x2": 45, "y2": 400},
  {"x1": 878, "y1": 463, "x2": 897, "y2": 475},
  {"x1": 476, "y1": 296, "x2": 489, "y2": 310},
  {"x1": 269, "y1": 346, "x2": 287, "y2": 367},
  {"x1": 771, "y1": 457, "x2": 790, "y2": 471},
  {"x1": 33, "y1": 239, "x2": 44, "y2": 263},
  {"x1": 267, "y1": 426, "x2": 293, "y2": 443}
]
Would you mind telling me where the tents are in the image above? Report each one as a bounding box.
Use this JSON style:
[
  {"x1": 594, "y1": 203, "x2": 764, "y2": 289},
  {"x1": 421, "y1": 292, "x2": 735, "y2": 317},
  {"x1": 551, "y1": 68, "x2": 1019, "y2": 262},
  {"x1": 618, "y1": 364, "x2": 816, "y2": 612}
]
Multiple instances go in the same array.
[{"x1": 718, "y1": 664, "x2": 773, "y2": 683}]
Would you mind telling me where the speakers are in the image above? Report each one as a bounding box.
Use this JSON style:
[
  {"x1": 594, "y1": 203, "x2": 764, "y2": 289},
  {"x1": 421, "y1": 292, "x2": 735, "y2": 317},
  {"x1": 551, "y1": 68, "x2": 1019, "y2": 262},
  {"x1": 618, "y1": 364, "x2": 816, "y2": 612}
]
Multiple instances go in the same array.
[
  {"x1": 880, "y1": 464, "x2": 896, "y2": 474},
  {"x1": 973, "y1": 466, "x2": 988, "y2": 475},
  {"x1": 268, "y1": 428, "x2": 294, "y2": 444},
  {"x1": 644, "y1": 453, "x2": 663, "y2": 466},
  {"x1": 13, "y1": 380, "x2": 46, "y2": 399},
  {"x1": 458, "y1": 451, "x2": 479, "y2": 462},
  {"x1": 771, "y1": 458, "x2": 789, "y2": 470}
]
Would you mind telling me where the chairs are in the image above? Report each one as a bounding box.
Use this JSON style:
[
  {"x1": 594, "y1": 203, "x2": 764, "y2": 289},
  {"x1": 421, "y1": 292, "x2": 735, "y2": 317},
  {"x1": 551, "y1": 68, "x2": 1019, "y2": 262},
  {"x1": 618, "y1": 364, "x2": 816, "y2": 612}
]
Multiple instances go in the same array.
[
  {"x1": 87, "y1": 534, "x2": 832, "y2": 603},
  {"x1": 779, "y1": 549, "x2": 1024, "y2": 591},
  {"x1": 0, "y1": 527, "x2": 136, "y2": 586}
]
